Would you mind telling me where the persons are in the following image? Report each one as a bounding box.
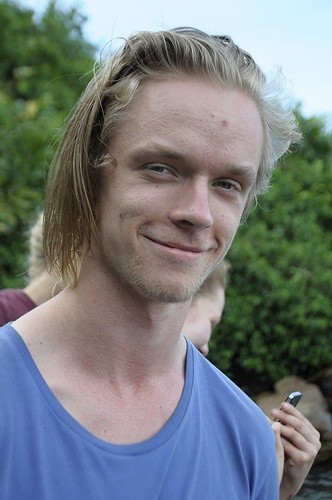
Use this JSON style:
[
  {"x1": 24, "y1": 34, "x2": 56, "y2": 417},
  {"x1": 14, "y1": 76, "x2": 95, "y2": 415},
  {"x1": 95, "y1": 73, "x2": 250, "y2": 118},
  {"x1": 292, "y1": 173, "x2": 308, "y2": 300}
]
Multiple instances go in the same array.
[
  {"x1": 0, "y1": 207, "x2": 323, "y2": 499},
  {"x1": 0, "y1": 25, "x2": 279, "y2": 499}
]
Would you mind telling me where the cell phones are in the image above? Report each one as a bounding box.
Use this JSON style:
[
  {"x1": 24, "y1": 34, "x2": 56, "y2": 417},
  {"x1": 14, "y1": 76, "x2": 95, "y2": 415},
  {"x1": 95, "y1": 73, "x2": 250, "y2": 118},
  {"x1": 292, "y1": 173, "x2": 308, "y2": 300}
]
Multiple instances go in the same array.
[{"x1": 273, "y1": 392, "x2": 303, "y2": 424}]
